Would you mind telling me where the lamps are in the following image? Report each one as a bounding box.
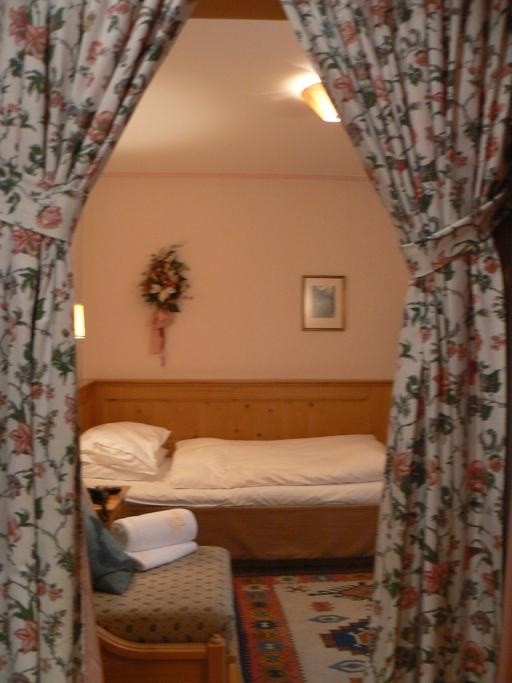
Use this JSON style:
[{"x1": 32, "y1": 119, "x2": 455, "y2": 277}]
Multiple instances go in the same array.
[
  {"x1": 299, "y1": 79, "x2": 342, "y2": 123},
  {"x1": 71, "y1": 300, "x2": 86, "y2": 340}
]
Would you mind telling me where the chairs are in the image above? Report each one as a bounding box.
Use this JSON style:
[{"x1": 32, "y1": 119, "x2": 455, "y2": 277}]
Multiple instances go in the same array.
[{"x1": 83, "y1": 529, "x2": 240, "y2": 683}]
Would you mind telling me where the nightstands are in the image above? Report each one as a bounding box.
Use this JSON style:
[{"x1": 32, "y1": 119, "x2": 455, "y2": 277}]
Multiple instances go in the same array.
[{"x1": 87, "y1": 484, "x2": 132, "y2": 530}]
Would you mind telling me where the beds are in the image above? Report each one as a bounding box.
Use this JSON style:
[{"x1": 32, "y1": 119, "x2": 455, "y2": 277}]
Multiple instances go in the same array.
[{"x1": 73, "y1": 418, "x2": 386, "y2": 573}]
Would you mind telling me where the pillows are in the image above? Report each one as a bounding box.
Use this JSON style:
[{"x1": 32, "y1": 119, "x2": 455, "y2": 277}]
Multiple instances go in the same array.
[{"x1": 78, "y1": 420, "x2": 174, "y2": 482}]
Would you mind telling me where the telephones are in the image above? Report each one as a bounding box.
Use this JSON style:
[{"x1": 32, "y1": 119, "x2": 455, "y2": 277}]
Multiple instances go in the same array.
[{"x1": 86, "y1": 488, "x2": 109, "y2": 505}]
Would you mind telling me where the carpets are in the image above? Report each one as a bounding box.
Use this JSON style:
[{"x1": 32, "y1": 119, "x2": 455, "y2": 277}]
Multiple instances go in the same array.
[{"x1": 227, "y1": 562, "x2": 380, "y2": 682}]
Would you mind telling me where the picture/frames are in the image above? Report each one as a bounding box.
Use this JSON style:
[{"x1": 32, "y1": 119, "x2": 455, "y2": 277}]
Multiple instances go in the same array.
[{"x1": 299, "y1": 272, "x2": 349, "y2": 333}]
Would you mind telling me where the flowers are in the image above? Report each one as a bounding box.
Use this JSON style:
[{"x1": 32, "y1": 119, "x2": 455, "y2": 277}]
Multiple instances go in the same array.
[{"x1": 133, "y1": 231, "x2": 196, "y2": 311}]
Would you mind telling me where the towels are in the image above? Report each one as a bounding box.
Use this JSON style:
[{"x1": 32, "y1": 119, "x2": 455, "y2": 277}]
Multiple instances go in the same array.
[{"x1": 106, "y1": 505, "x2": 200, "y2": 572}]
